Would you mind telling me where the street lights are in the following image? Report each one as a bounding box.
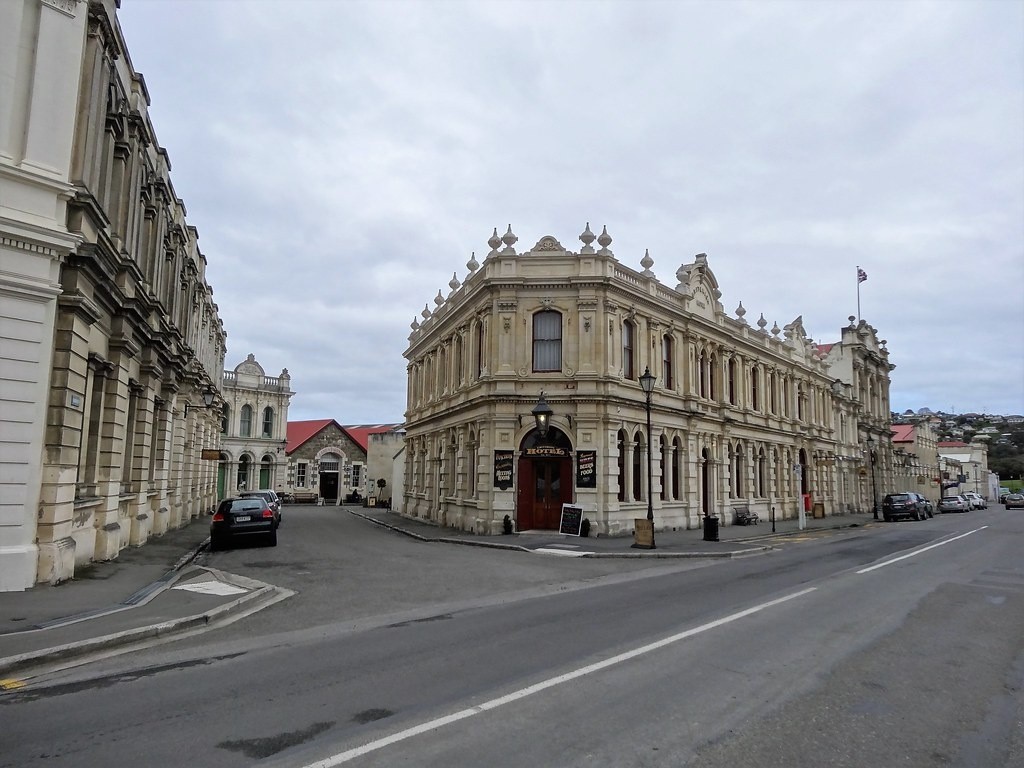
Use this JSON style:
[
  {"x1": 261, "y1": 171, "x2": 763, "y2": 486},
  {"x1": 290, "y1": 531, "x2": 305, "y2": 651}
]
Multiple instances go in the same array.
[
  {"x1": 637, "y1": 366, "x2": 657, "y2": 549},
  {"x1": 973, "y1": 463, "x2": 979, "y2": 494},
  {"x1": 935, "y1": 452, "x2": 943, "y2": 499},
  {"x1": 866, "y1": 435, "x2": 878, "y2": 519}
]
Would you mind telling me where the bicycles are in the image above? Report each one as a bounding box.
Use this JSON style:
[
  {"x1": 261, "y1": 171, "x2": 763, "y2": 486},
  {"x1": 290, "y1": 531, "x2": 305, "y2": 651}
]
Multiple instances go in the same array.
[{"x1": 281, "y1": 492, "x2": 296, "y2": 504}]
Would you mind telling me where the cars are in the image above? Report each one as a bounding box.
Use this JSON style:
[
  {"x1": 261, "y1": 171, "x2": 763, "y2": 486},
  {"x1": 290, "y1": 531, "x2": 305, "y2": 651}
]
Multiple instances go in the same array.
[
  {"x1": 1006, "y1": 495, "x2": 1024, "y2": 510},
  {"x1": 1000, "y1": 493, "x2": 1010, "y2": 503},
  {"x1": 915, "y1": 493, "x2": 934, "y2": 518},
  {"x1": 882, "y1": 493, "x2": 927, "y2": 522},
  {"x1": 938, "y1": 495, "x2": 971, "y2": 514},
  {"x1": 959, "y1": 492, "x2": 988, "y2": 511},
  {"x1": 208, "y1": 497, "x2": 278, "y2": 553},
  {"x1": 239, "y1": 489, "x2": 282, "y2": 529}
]
